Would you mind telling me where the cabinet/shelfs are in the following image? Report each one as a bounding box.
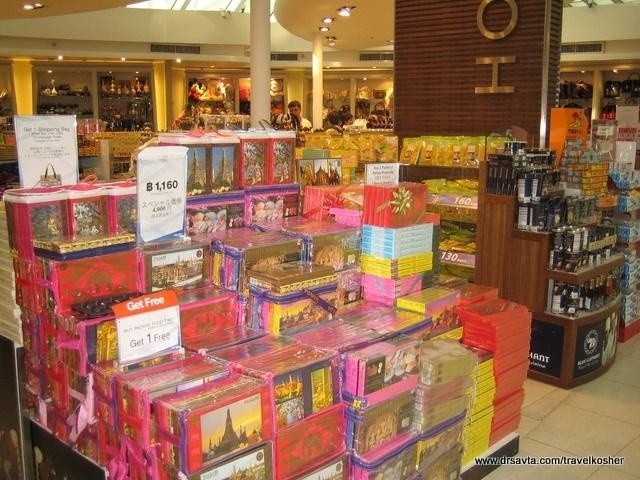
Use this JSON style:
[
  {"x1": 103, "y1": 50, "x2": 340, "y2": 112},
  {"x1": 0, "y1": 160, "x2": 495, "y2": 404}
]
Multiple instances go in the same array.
[{"x1": 474, "y1": 161, "x2": 628, "y2": 391}]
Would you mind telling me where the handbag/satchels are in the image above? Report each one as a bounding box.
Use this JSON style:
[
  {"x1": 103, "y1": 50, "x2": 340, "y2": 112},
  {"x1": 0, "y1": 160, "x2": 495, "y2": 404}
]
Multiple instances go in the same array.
[
  {"x1": 272, "y1": 114, "x2": 299, "y2": 128},
  {"x1": 604, "y1": 79, "x2": 640, "y2": 98},
  {"x1": 367, "y1": 109, "x2": 395, "y2": 129},
  {"x1": 560, "y1": 80, "x2": 592, "y2": 98}
]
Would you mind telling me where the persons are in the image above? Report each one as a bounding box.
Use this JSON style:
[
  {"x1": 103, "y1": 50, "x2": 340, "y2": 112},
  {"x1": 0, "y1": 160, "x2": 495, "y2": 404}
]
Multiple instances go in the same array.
[
  {"x1": 287, "y1": 101, "x2": 313, "y2": 130},
  {"x1": 325, "y1": 104, "x2": 354, "y2": 132}
]
